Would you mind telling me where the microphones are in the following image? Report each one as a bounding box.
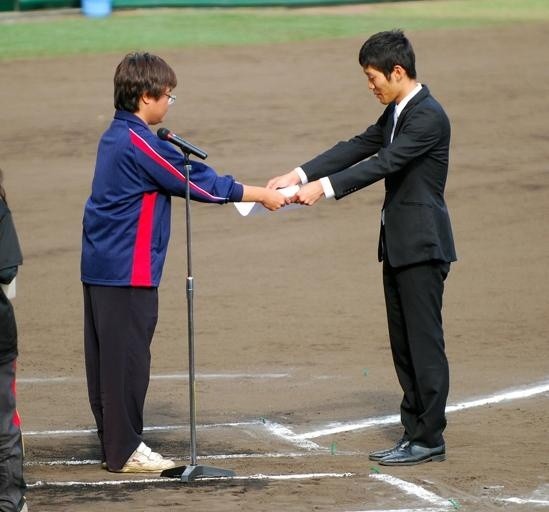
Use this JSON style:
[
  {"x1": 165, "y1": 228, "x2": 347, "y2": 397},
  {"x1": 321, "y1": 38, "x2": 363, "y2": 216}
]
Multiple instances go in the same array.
[{"x1": 157, "y1": 128, "x2": 207, "y2": 159}]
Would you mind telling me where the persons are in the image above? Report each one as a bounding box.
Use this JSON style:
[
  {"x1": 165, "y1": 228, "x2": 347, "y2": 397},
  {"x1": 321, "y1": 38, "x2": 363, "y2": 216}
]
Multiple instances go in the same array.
[
  {"x1": 266, "y1": 28, "x2": 458, "y2": 467},
  {"x1": 79, "y1": 50, "x2": 293, "y2": 475},
  {"x1": 0, "y1": 171, "x2": 27, "y2": 512}
]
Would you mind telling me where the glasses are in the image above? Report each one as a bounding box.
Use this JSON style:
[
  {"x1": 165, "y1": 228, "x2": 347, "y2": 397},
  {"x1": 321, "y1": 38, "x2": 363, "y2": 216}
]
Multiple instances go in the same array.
[{"x1": 162, "y1": 91, "x2": 177, "y2": 105}]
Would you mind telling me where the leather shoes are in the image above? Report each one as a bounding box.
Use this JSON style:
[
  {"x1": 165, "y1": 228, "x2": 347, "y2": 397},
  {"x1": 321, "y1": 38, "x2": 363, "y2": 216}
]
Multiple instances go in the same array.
[
  {"x1": 368, "y1": 437, "x2": 411, "y2": 461},
  {"x1": 378, "y1": 442, "x2": 446, "y2": 467}
]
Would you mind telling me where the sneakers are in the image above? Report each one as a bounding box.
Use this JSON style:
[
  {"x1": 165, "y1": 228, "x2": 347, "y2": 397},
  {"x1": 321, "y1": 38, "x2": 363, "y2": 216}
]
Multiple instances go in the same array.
[{"x1": 110, "y1": 442, "x2": 176, "y2": 473}]
